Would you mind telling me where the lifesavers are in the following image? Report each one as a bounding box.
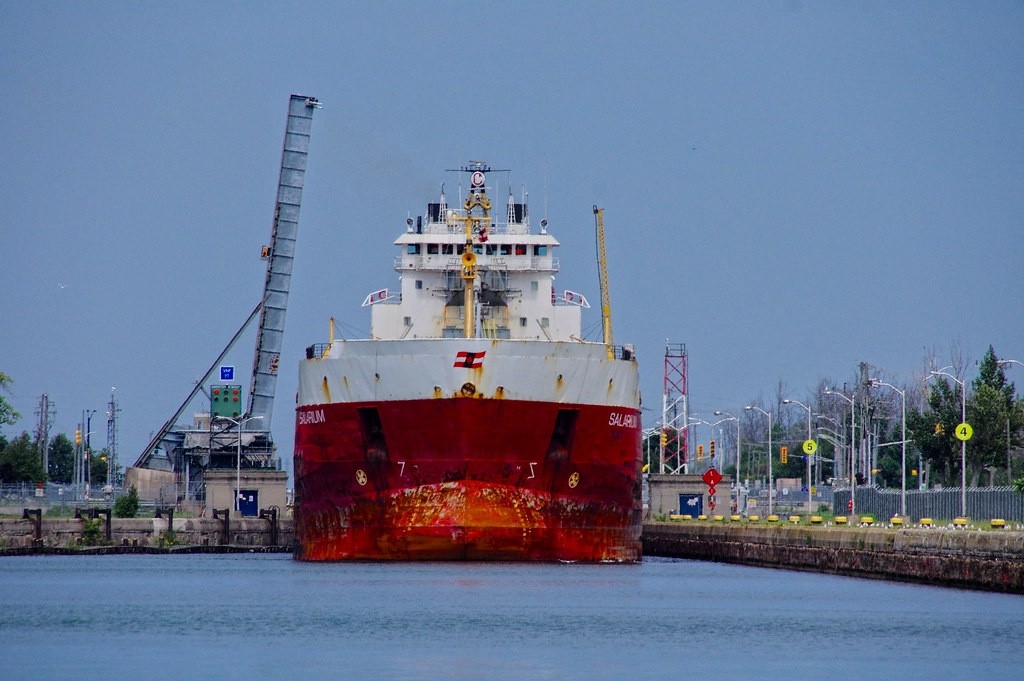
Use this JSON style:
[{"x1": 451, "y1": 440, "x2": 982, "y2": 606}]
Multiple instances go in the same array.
[
  {"x1": 562, "y1": 292, "x2": 574, "y2": 301},
  {"x1": 378, "y1": 290, "x2": 389, "y2": 300}
]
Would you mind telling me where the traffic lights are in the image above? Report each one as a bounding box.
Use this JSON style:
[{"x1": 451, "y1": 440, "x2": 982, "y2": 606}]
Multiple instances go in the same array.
[
  {"x1": 710, "y1": 440, "x2": 715, "y2": 458},
  {"x1": 782, "y1": 447, "x2": 787, "y2": 463},
  {"x1": 698, "y1": 445, "x2": 703, "y2": 457},
  {"x1": 662, "y1": 434, "x2": 667, "y2": 446}
]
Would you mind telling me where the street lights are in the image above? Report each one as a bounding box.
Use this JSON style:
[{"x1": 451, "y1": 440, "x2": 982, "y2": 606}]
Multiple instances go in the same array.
[
  {"x1": 688, "y1": 416, "x2": 736, "y2": 470},
  {"x1": 929, "y1": 370, "x2": 967, "y2": 517},
  {"x1": 713, "y1": 411, "x2": 739, "y2": 514},
  {"x1": 823, "y1": 391, "x2": 854, "y2": 516},
  {"x1": 657, "y1": 421, "x2": 701, "y2": 474},
  {"x1": 641, "y1": 427, "x2": 661, "y2": 476},
  {"x1": 216, "y1": 415, "x2": 263, "y2": 510},
  {"x1": 862, "y1": 381, "x2": 906, "y2": 517},
  {"x1": 744, "y1": 406, "x2": 772, "y2": 516},
  {"x1": 782, "y1": 399, "x2": 812, "y2": 516},
  {"x1": 76, "y1": 432, "x2": 97, "y2": 501}
]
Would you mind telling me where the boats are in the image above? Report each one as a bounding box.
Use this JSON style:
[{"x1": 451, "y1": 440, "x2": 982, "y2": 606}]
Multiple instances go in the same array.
[{"x1": 292, "y1": 162, "x2": 644, "y2": 563}]
